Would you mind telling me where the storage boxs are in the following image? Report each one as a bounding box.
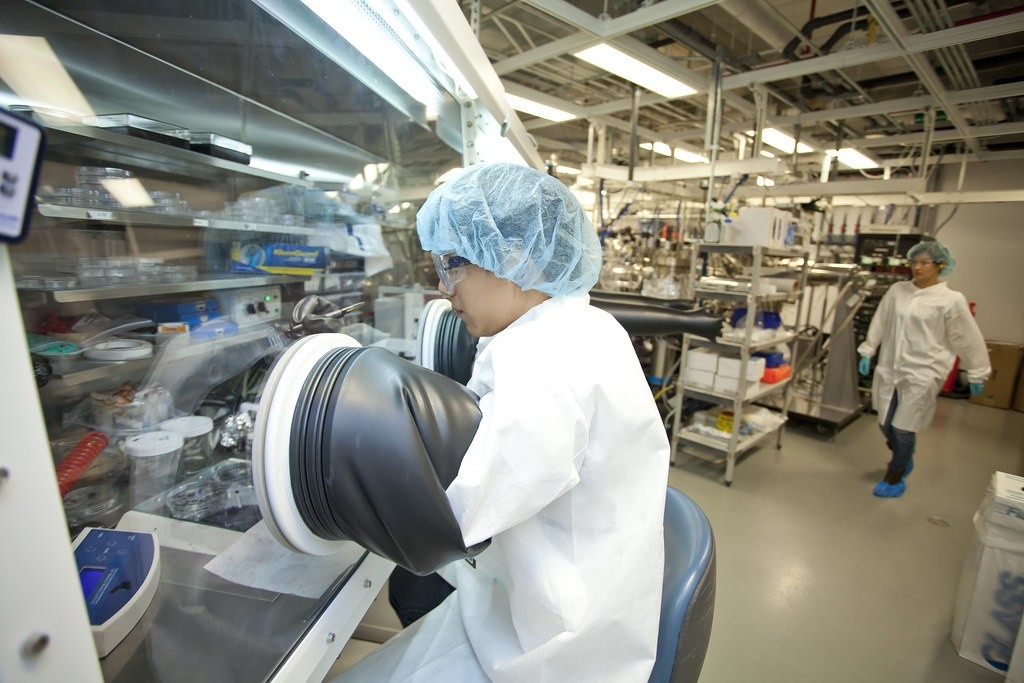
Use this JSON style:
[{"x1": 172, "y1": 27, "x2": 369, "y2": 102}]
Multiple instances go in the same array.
[{"x1": 948, "y1": 472, "x2": 1024, "y2": 673}]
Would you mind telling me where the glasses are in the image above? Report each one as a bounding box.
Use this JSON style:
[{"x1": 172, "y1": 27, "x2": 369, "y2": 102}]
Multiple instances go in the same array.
[
  {"x1": 432, "y1": 250, "x2": 469, "y2": 289},
  {"x1": 907, "y1": 258, "x2": 934, "y2": 268}
]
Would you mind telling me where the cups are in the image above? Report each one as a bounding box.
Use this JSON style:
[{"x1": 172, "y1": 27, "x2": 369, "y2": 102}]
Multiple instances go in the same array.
[
  {"x1": 127, "y1": 430, "x2": 185, "y2": 495},
  {"x1": 160, "y1": 413, "x2": 217, "y2": 475}
]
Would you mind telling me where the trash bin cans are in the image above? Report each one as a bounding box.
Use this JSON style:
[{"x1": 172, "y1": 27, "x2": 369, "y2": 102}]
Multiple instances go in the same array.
[{"x1": 948, "y1": 471, "x2": 1024, "y2": 676}]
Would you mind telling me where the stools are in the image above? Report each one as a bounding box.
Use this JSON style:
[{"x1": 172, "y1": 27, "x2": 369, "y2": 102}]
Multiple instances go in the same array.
[{"x1": 649, "y1": 483, "x2": 719, "y2": 683}]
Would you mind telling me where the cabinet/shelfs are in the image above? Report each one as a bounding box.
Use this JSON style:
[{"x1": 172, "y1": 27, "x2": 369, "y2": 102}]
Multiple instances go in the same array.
[
  {"x1": 667, "y1": 236, "x2": 812, "y2": 488},
  {"x1": 3, "y1": 102, "x2": 367, "y2": 402}
]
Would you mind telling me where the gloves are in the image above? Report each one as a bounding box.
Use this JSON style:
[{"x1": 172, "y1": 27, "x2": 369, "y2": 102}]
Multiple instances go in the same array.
[
  {"x1": 970, "y1": 381, "x2": 986, "y2": 397},
  {"x1": 859, "y1": 357, "x2": 869, "y2": 375}
]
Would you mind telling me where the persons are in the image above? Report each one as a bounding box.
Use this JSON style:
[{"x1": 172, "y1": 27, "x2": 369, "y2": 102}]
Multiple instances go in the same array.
[
  {"x1": 855, "y1": 238, "x2": 993, "y2": 501},
  {"x1": 279, "y1": 162, "x2": 671, "y2": 683}
]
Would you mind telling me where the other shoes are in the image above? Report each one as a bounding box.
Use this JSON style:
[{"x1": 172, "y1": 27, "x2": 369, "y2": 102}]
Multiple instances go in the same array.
[{"x1": 875, "y1": 460, "x2": 914, "y2": 497}]
